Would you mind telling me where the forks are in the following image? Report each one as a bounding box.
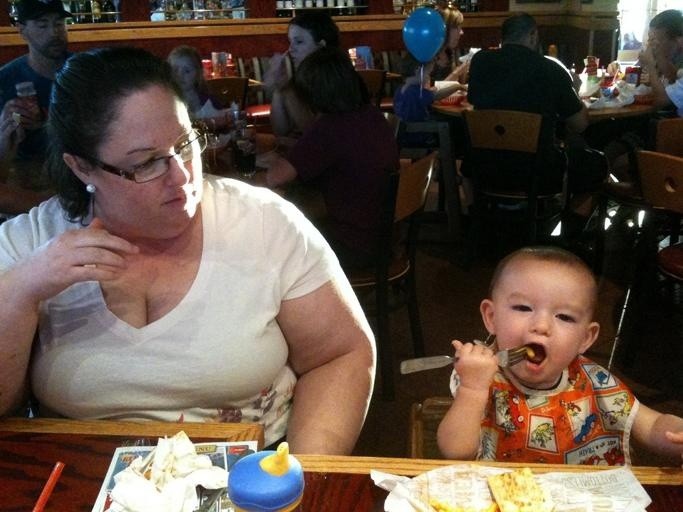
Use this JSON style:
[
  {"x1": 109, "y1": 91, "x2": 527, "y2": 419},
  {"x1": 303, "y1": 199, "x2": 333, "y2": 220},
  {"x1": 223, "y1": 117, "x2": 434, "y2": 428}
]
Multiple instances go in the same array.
[{"x1": 398, "y1": 342, "x2": 529, "y2": 377}]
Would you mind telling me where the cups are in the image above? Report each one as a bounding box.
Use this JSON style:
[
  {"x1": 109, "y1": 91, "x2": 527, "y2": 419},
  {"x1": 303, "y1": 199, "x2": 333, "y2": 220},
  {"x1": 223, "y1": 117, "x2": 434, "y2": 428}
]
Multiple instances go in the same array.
[{"x1": 224, "y1": 109, "x2": 259, "y2": 178}]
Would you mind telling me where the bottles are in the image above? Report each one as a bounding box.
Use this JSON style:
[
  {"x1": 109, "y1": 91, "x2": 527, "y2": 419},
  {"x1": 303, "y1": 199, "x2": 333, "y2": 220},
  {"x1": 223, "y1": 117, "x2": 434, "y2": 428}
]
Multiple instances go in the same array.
[
  {"x1": 12, "y1": 80, "x2": 54, "y2": 193},
  {"x1": 64, "y1": 0, "x2": 123, "y2": 23},
  {"x1": 228, "y1": 438, "x2": 306, "y2": 512}
]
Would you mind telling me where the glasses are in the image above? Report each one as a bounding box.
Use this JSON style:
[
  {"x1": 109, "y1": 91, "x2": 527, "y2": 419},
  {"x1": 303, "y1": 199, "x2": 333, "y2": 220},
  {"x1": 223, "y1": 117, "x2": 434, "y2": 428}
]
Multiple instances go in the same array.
[{"x1": 90, "y1": 129, "x2": 208, "y2": 183}]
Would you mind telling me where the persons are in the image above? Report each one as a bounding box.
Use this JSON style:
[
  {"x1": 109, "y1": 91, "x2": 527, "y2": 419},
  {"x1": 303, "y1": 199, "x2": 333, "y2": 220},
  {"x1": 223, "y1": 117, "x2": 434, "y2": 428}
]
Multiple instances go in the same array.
[
  {"x1": 398, "y1": 4, "x2": 471, "y2": 161},
  {"x1": 264, "y1": 12, "x2": 340, "y2": 137},
  {"x1": 0, "y1": 1, "x2": 77, "y2": 160},
  {"x1": 636, "y1": 10, "x2": 683, "y2": 151},
  {"x1": 167, "y1": 46, "x2": 221, "y2": 114},
  {"x1": 393, "y1": 54, "x2": 471, "y2": 148},
  {"x1": 83, "y1": 0, "x2": 229, "y2": 23},
  {"x1": 466, "y1": 10, "x2": 610, "y2": 256},
  {"x1": 0, "y1": 44, "x2": 378, "y2": 455},
  {"x1": 0, "y1": 113, "x2": 54, "y2": 216},
  {"x1": 435, "y1": 243, "x2": 682, "y2": 467},
  {"x1": 252, "y1": 47, "x2": 400, "y2": 271}
]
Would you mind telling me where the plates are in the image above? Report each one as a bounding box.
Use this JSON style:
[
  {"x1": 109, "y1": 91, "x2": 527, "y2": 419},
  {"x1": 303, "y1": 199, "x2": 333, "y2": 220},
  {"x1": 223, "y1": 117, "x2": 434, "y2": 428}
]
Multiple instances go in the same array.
[{"x1": 382, "y1": 463, "x2": 516, "y2": 512}]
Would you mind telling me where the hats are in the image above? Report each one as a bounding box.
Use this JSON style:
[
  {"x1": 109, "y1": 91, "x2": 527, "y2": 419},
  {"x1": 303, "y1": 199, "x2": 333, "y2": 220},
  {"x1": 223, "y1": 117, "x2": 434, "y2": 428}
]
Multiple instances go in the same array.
[{"x1": 10, "y1": 0, "x2": 74, "y2": 20}]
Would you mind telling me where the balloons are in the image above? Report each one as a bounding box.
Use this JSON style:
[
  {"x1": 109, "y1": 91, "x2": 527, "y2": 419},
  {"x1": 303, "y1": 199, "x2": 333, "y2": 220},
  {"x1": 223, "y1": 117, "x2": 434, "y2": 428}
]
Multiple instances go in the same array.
[{"x1": 402, "y1": 7, "x2": 445, "y2": 65}]
